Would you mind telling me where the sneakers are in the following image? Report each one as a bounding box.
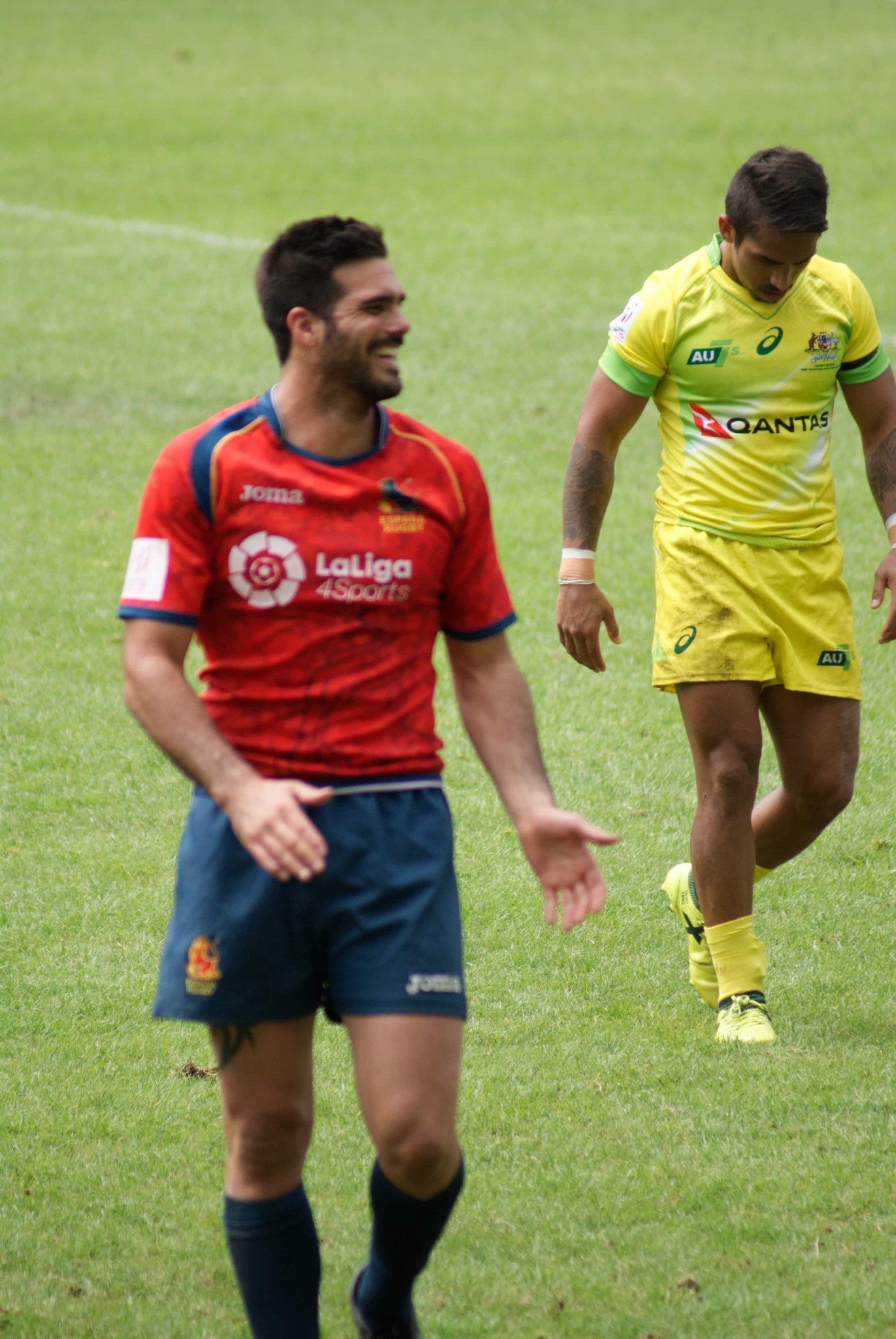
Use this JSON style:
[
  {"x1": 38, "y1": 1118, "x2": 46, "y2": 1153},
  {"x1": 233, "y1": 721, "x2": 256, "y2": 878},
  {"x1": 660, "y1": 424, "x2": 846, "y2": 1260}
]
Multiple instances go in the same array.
[
  {"x1": 659, "y1": 862, "x2": 719, "y2": 1011},
  {"x1": 348, "y1": 1265, "x2": 421, "y2": 1339},
  {"x1": 714, "y1": 995, "x2": 775, "y2": 1044}
]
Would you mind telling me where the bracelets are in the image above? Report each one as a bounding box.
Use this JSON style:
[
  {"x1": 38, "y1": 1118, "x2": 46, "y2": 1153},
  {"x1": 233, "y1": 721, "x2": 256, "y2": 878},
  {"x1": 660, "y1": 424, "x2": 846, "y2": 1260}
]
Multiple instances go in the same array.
[
  {"x1": 884, "y1": 512, "x2": 896, "y2": 550},
  {"x1": 558, "y1": 548, "x2": 595, "y2": 585}
]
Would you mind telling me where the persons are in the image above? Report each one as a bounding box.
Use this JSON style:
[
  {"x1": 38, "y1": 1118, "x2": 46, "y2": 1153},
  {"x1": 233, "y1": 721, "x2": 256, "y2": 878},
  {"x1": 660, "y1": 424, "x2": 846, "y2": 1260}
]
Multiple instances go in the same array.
[
  {"x1": 119, "y1": 214, "x2": 617, "y2": 1339},
  {"x1": 558, "y1": 146, "x2": 896, "y2": 1049}
]
